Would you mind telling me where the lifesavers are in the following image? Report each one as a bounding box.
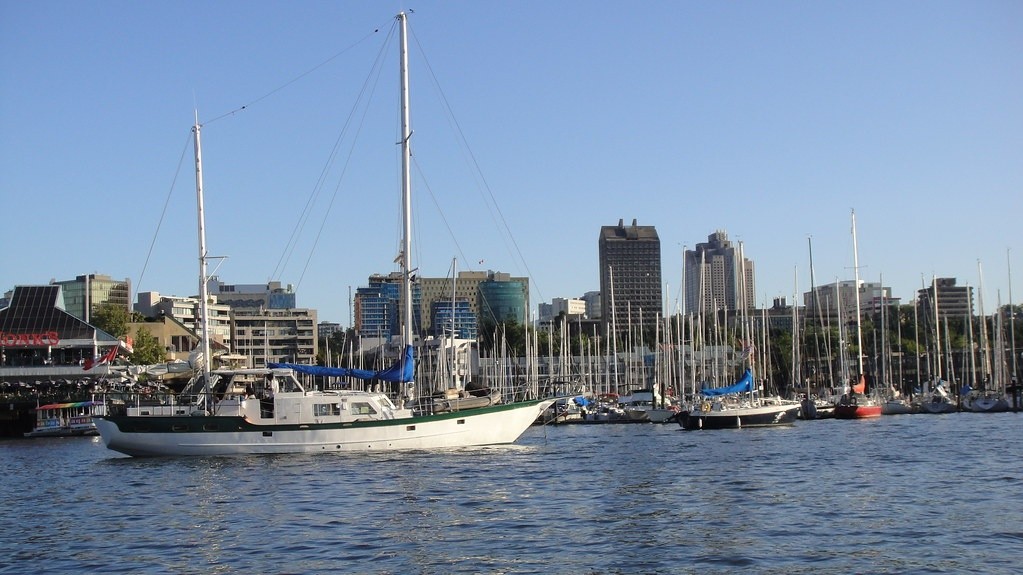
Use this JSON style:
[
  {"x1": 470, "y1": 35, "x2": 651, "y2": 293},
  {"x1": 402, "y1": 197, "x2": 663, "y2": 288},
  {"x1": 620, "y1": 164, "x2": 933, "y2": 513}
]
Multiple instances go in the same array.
[{"x1": 702, "y1": 402, "x2": 710, "y2": 412}]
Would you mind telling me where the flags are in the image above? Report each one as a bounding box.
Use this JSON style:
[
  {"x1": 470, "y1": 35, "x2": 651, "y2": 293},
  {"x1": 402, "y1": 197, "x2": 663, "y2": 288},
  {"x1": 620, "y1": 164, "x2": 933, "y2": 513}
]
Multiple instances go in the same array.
[
  {"x1": 84, "y1": 346, "x2": 117, "y2": 369},
  {"x1": 741, "y1": 347, "x2": 753, "y2": 359}
]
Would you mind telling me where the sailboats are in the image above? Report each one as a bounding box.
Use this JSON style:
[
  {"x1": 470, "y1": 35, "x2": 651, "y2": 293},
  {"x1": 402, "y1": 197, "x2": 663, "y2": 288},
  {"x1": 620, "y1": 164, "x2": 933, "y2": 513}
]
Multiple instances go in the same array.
[
  {"x1": 91, "y1": 11, "x2": 593, "y2": 457},
  {"x1": 412, "y1": 207, "x2": 1023, "y2": 430}
]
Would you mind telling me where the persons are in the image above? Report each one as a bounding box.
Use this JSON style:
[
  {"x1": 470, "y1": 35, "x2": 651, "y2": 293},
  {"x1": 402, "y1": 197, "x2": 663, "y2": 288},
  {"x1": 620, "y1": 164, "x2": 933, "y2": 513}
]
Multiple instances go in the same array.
[{"x1": 246, "y1": 387, "x2": 255, "y2": 396}]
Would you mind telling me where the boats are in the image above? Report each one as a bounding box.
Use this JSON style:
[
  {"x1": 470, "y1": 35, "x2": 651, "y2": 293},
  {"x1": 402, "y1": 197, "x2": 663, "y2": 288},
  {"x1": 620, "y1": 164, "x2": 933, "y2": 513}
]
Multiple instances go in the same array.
[{"x1": 24, "y1": 401, "x2": 108, "y2": 437}]
